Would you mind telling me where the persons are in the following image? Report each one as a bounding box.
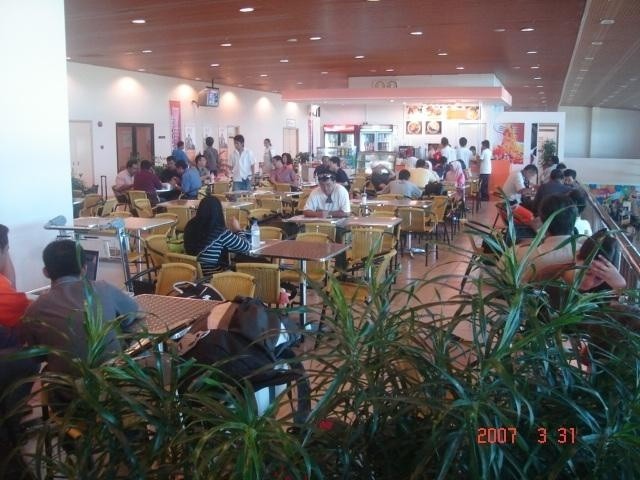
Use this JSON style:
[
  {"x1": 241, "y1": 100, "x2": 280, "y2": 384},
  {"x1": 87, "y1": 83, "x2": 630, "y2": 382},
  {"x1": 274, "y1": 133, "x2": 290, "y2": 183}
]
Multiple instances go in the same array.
[
  {"x1": 521, "y1": 224, "x2": 630, "y2": 376},
  {"x1": 224, "y1": 135, "x2": 256, "y2": 192},
  {"x1": 573, "y1": 303, "x2": 640, "y2": 377},
  {"x1": 1, "y1": 222, "x2": 43, "y2": 443},
  {"x1": 184, "y1": 195, "x2": 250, "y2": 274},
  {"x1": 262, "y1": 139, "x2": 296, "y2": 194},
  {"x1": 114, "y1": 137, "x2": 220, "y2": 216},
  {"x1": 370, "y1": 137, "x2": 495, "y2": 199},
  {"x1": 18, "y1": 240, "x2": 148, "y2": 458},
  {"x1": 303, "y1": 156, "x2": 350, "y2": 280},
  {"x1": 558, "y1": 322, "x2": 584, "y2": 363},
  {"x1": 502, "y1": 155, "x2": 586, "y2": 235}
]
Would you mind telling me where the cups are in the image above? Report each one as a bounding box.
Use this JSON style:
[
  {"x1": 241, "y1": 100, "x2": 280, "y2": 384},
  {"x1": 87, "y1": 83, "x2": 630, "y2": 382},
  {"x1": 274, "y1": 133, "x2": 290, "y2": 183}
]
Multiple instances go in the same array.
[{"x1": 362, "y1": 193, "x2": 367, "y2": 204}]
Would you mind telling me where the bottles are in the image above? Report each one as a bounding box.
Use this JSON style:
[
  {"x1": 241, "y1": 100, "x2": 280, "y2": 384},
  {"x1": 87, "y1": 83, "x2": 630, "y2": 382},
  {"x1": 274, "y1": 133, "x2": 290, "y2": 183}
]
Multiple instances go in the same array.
[
  {"x1": 250, "y1": 220, "x2": 261, "y2": 249},
  {"x1": 210, "y1": 172, "x2": 215, "y2": 183}
]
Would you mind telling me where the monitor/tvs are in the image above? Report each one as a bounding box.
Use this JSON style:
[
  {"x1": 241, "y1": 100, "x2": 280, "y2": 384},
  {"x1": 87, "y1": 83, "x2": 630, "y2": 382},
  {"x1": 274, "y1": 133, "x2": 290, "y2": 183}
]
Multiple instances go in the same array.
[{"x1": 197, "y1": 87, "x2": 220, "y2": 108}]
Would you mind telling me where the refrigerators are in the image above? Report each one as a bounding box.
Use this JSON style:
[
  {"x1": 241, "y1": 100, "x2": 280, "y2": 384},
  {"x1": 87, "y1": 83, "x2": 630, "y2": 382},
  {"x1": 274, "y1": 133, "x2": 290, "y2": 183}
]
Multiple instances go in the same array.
[
  {"x1": 360, "y1": 126, "x2": 392, "y2": 162},
  {"x1": 324, "y1": 125, "x2": 359, "y2": 166}
]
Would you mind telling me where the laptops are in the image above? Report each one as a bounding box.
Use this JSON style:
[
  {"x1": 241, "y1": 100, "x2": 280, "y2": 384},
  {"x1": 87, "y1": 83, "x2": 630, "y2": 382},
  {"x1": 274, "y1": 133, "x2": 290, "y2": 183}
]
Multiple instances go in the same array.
[{"x1": 29, "y1": 249, "x2": 99, "y2": 299}]
